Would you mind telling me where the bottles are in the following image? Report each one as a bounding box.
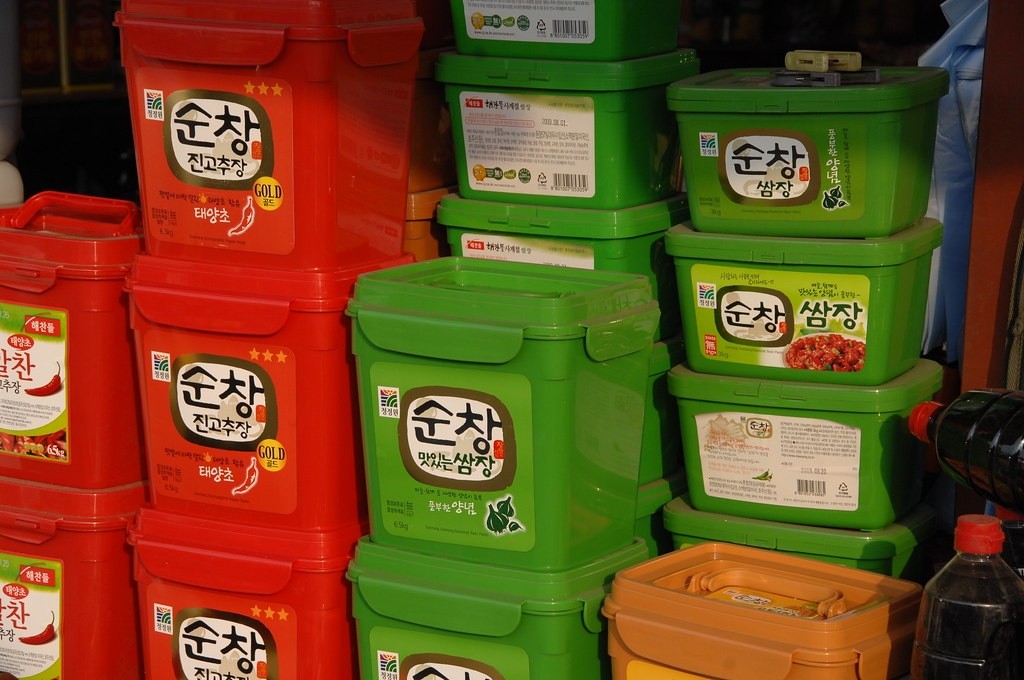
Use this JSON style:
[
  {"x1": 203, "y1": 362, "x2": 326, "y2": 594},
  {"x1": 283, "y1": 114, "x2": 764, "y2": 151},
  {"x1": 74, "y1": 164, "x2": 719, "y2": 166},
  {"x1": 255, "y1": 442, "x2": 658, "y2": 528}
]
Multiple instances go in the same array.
[
  {"x1": 906, "y1": 512, "x2": 1024, "y2": 680},
  {"x1": 908, "y1": 386, "x2": 1024, "y2": 519}
]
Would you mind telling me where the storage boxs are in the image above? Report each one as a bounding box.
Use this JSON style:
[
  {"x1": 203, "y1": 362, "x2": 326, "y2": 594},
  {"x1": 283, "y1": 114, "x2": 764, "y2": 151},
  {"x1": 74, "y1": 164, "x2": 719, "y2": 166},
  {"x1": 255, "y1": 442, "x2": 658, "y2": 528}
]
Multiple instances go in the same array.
[
  {"x1": 601, "y1": 542, "x2": 920, "y2": 680},
  {"x1": 436, "y1": 48, "x2": 700, "y2": 211},
  {"x1": 0, "y1": 0, "x2": 451, "y2": 680},
  {"x1": 343, "y1": 256, "x2": 658, "y2": 572},
  {"x1": 344, "y1": 535, "x2": 647, "y2": 680},
  {"x1": 435, "y1": 191, "x2": 688, "y2": 344},
  {"x1": 663, "y1": 494, "x2": 918, "y2": 578},
  {"x1": 668, "y1": 357, "x2": 943, "y2": 533},
  {"x1": 666, "y1": 68, "x2": 950, "y2": 237},
  {"x1": 665, "y1": 219, "x2": 942, "y2": 384},
  {"x1": 451, "y1": 0, "x2": 678, "y2": 61}
]
